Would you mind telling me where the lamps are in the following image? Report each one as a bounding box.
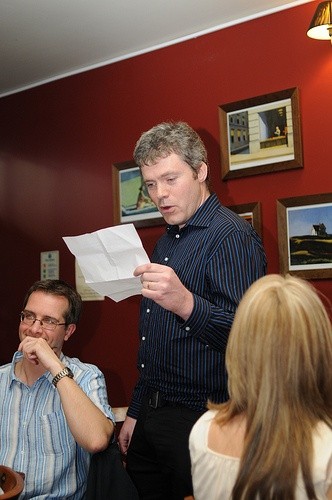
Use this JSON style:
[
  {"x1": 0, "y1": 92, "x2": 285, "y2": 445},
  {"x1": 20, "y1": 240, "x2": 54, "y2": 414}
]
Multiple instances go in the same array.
[{"x1": 306, "y1": 0, "x2": 332, "y2": 45}]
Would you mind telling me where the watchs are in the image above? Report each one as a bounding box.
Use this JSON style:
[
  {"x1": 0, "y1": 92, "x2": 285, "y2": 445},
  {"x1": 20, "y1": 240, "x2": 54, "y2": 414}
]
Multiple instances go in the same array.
[{"x1": 52, "y1": 367, "x2": 74, "y2": 388}]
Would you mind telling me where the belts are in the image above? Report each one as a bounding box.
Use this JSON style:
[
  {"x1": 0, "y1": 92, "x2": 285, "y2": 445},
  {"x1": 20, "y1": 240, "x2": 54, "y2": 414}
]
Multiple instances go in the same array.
[{"x1": 147, "y1": 391, "x2": 201, "y2": 411}]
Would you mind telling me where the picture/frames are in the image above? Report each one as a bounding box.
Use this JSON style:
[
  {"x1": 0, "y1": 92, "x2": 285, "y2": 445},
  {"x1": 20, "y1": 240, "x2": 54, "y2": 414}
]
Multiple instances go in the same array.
[
  {"x1": 216, "y1": 86, "x2": 304, "y2": 182},
  {"x1": 276, "y1": 193, "x2": 332, "y2": 280},
  {"x1": 111, "y1": 159, "x2": 167, "y2": 229},
  {"x1": 226, "y1": 202, "x2": 263, "y2": 240}
]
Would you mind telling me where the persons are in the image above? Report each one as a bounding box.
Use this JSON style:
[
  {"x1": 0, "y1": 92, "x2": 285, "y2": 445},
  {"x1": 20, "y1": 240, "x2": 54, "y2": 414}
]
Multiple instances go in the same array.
[
  {"x1": 0, "y1": 280, "x2": 115, "y2": 500},
  {"x1": 136, "y1": 185, "x2": 156, "y2": 210},
  {"x1": 189, "y1": 274, "x2": 332, "y2": 500},
  {"x1": 119, "y1": 121, "x2": 266, "y2": 500}
]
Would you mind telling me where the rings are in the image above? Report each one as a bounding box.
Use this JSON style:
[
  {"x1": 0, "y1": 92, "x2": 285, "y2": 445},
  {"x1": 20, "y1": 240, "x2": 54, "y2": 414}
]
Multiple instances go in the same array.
[{"x1": 146, "y1": 281, "x2": 152, "y2": 289}]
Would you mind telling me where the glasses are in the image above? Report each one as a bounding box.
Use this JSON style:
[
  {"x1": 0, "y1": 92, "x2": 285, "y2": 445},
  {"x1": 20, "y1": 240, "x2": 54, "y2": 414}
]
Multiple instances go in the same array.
[{"x1": 20, "y1": 311, "x2": 69, "y2": 330}]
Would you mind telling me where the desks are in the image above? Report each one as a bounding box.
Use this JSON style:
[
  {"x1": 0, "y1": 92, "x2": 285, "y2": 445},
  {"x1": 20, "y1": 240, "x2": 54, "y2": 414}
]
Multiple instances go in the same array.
[{"x1": 86, "y1": 441, "x2": 140, "y2": 500}]
